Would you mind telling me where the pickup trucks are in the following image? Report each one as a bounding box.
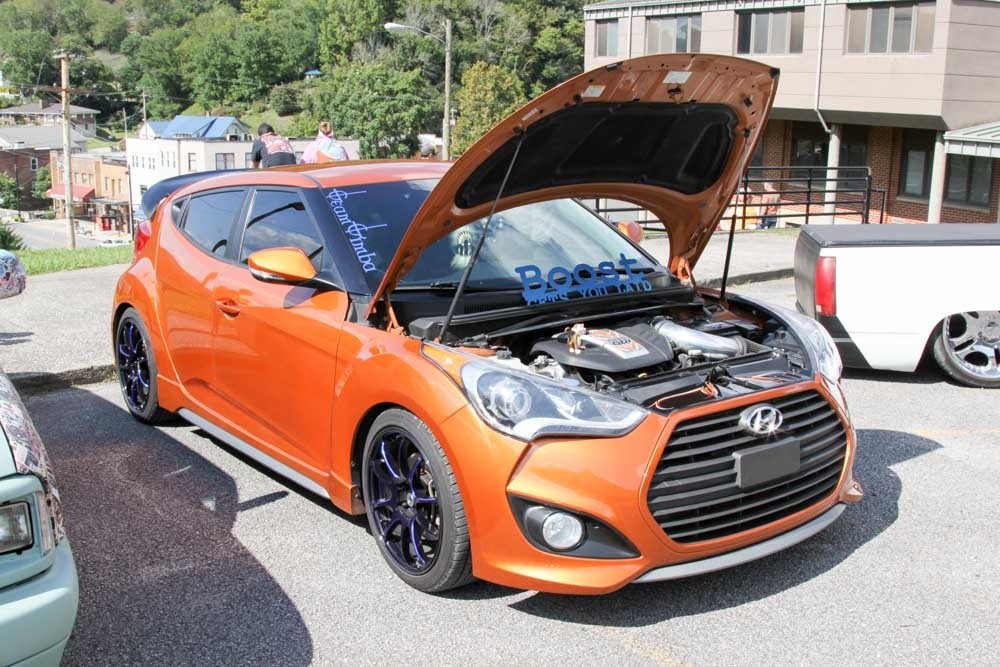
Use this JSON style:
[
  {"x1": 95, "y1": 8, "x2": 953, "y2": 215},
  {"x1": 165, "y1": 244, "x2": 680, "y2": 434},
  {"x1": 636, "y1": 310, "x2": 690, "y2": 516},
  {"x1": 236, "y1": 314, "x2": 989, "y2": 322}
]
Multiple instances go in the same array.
[{"x1": 794, "y1": 222, "x2": 1000, "y2": 393}]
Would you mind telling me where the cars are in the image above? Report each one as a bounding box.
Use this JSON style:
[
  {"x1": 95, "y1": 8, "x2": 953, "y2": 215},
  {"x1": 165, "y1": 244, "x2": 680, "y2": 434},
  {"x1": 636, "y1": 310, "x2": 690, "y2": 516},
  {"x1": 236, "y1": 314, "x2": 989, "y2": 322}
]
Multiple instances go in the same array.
[
  {"x1": 128, "y1": 166, "x2": 454, "y2": 302},
  {"x1": 0, "y1": 368, "x2": 80, "y2": 666},
  {"x1": 110, "y1": 52, "x2": 866, "y2": 594}
]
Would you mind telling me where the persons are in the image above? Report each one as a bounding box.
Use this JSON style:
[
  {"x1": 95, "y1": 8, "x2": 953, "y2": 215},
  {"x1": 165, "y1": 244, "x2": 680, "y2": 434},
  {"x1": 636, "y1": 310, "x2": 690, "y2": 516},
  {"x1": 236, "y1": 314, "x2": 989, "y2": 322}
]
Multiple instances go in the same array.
[
  {"x1": 298, "y1": 118, "x2": 349, "y2": 164},
  {"x1": 420, "y1": 142, "x2": 439, "y2": 161},
  {"x1": 251, "y1": 123, "x2": 296, "y2": 169},
  {"x1": 714, "y1": 178, "x2": 781, "y2": 231}
]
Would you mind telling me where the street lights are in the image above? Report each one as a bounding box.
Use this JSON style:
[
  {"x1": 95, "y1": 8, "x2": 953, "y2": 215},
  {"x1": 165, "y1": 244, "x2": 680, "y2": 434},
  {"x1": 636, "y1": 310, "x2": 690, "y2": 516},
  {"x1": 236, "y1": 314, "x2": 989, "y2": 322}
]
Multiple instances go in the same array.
[{"x1": 382, "y1": 17, "x2": 453, "y2": 163}]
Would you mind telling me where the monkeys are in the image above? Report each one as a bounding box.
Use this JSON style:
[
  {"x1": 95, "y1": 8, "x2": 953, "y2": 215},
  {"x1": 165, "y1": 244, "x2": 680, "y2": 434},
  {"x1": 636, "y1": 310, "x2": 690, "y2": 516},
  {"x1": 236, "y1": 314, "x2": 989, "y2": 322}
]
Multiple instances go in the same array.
[{"x1": 564, "y1": 323, "x2": 587, "y2": 354}]
